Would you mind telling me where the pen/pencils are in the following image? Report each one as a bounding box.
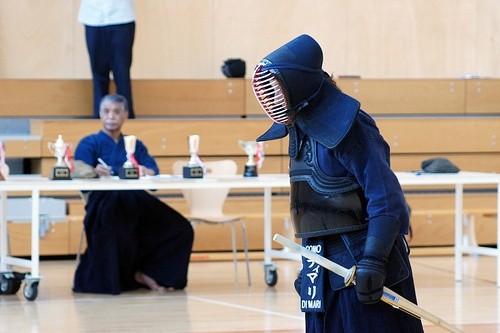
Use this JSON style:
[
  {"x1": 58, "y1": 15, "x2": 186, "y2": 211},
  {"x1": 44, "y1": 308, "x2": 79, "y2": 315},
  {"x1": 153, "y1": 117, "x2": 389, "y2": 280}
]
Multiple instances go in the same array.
[{"x1": 97, "y1": 157, "x2": 113, "y2": 174}]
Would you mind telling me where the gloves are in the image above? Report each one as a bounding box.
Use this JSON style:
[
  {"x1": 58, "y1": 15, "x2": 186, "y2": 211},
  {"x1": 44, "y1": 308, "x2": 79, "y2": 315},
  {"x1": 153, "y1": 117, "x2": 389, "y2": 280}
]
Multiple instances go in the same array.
[
  {"x1": 294, "y1": 278, "x2": 302, "y2": 295},
  {"x1": 354, "y1": 263, "x2": 387, "y2": 306}
]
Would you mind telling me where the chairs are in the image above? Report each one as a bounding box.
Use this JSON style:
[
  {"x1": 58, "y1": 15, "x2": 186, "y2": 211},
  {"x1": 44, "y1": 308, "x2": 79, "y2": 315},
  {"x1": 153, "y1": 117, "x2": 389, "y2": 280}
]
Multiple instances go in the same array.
[{"x1": 174, "y1": 160, "x2": 244, "y2": 288}]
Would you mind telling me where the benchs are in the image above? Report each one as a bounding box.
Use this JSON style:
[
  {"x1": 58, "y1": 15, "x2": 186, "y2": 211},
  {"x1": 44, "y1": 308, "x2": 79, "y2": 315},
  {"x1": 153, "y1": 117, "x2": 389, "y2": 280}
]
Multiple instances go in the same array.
[{"x1": 1, "y1": 76, "x2": 500, "y2": 258}]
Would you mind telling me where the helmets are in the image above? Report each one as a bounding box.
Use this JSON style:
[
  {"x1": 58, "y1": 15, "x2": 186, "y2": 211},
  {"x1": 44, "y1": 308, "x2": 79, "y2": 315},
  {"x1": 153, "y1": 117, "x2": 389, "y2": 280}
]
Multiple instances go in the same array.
[{"x1": 252, "y1": 34, "x2": 326, "y2": 125}]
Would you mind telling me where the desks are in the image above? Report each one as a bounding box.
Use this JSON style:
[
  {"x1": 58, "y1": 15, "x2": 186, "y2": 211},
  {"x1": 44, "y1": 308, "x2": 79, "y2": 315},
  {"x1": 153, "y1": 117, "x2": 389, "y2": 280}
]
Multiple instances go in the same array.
[{"x1": 0, "y1": 174, "x2": 499, "y2": 300}]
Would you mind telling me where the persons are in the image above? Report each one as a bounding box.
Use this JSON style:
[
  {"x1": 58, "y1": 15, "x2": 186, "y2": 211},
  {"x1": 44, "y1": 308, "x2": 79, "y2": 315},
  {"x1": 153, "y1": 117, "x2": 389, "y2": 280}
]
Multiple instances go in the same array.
[
  {"x1": 253, "y1": 35, "x2": 425, "y2": 333},
  {"x1": 80, "y1": 0, "x2": 139, "y2": 120},
  {"x1": 71, "y1": 95, "x2": 195, "y2": 293}
]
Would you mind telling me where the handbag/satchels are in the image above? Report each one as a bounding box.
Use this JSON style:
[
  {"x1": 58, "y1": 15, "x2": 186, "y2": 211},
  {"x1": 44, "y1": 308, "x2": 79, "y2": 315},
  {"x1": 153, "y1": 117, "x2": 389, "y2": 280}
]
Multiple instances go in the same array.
[{"x1": 421, "y1": 158, "x2": 459, "y2": 174}]
